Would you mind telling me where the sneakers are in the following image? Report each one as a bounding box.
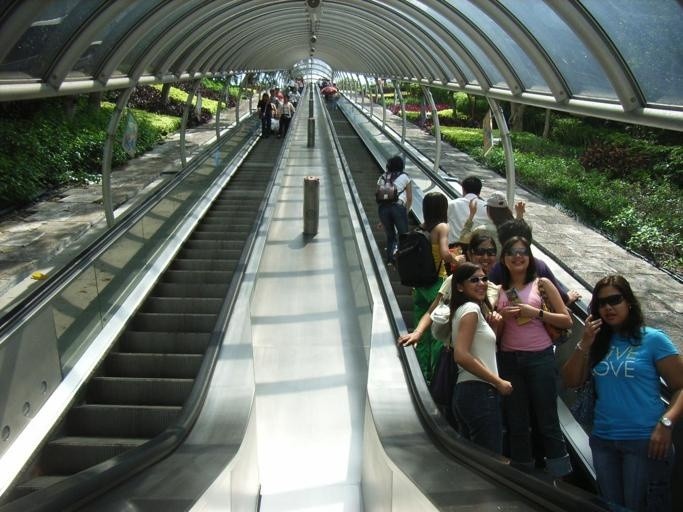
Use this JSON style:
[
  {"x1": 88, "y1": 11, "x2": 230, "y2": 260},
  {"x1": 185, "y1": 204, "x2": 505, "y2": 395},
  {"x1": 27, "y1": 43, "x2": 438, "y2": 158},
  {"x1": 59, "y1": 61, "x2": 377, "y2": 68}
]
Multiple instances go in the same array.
[
  {"x1": 387, "y1": 262, "x2": 395, "y2": 273},
  {"x1": 393, "y1": 249, "x2": 398, "y2": 256}
]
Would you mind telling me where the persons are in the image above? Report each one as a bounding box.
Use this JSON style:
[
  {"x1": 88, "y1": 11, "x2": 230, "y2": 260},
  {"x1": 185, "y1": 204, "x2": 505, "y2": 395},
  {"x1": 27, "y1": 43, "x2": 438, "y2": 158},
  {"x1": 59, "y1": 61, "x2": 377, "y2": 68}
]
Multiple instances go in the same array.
[
  {"x1": 315, "y1": 78, "x2": 339, "y2": 113},
  {"x1": 377, "y1": 156, "x2": 581, "y2": 486},
  {"x1": 560, "y1": 273, "x2": 682, "y2": 511},
  {"x1": 257, "y1": 77, "x2": 303, "y2": 138}
]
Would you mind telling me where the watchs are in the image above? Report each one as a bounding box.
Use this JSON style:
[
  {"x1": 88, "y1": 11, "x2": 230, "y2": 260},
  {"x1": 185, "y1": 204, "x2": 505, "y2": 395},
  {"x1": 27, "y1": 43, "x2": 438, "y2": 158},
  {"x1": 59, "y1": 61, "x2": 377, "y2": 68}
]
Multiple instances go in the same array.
[{"x1": 658, "y1": 415, "x2": 675, "y2": 431}]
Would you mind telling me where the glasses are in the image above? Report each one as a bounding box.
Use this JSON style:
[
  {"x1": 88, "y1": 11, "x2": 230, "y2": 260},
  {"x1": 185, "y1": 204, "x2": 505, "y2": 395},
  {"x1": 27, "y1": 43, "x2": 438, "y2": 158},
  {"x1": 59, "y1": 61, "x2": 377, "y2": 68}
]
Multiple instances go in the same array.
[
  {"x1": 471, "y1": 247, "x2": 496, "y2": 256},
  {"x1": 467, "y1": 277, "x2": 487, "y2": 282},
  {"x1": 503, "y1": 248, "x2": 529, "y2": 256},
  {"x1": 596, "y1": 295, "x2": 624, "y2": 308}
]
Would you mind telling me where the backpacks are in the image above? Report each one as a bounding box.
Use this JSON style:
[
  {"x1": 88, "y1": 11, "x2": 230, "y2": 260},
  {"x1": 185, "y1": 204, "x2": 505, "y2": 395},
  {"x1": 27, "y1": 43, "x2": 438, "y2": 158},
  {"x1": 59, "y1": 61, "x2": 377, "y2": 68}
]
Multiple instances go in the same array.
[
  {"x1": 376, "y1": 171, "x2": 408, "y2": 203},
  {"x1": 396, "y1": 222, "x2": 442, "y2": 287}
]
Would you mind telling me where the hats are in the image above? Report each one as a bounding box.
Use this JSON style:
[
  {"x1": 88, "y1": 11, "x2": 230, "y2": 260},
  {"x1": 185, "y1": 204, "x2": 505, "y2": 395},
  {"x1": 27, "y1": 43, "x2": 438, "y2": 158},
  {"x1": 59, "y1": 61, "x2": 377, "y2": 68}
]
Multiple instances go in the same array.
[{"x1": 483, "y1": 191, "x2": 507, "y2": 208}]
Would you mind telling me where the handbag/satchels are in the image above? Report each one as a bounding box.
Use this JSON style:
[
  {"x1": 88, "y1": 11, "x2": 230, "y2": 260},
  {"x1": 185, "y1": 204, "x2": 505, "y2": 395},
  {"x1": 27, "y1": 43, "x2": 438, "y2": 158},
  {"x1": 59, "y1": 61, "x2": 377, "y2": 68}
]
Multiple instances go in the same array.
[
  {"x1": 430, "y1": 345, "x2": 458, "y2": 406},
  {"x1": 430, "y1": 301, "x2": 451, "y2": 342},
  {"x1": 270, "y1": 117, "x2": 279, "y2": 132},
  {"x1": 569, "y1": 374, "x2": 595, "y2": 425},
  {"x1": 538, "y1": 277, "x2": 576, "y2": 346},
  {"x1": 259, "y1": 112, "x2": 266, "y2": 119}
]
